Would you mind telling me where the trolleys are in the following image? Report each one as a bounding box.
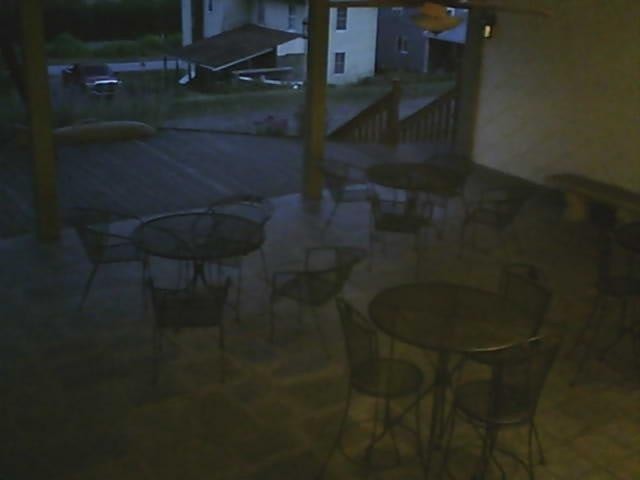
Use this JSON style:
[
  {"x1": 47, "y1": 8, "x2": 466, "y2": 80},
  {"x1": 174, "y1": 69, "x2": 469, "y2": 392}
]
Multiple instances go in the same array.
[{"x1": 321, "y1": 0, "x2": 551, "y2": 19}]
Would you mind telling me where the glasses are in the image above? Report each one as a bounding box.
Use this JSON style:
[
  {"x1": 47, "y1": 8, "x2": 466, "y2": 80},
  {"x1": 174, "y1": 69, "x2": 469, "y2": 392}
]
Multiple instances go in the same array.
[
  {"x1": 479, "y1": 15, "x2": 494, "y2": 38},
  {"x1": 410, "y1": 5, "x2": 462, "y2": 35}
]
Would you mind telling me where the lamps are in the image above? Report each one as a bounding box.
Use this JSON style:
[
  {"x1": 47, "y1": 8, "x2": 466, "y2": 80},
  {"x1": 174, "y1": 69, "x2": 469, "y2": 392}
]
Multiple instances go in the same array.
[
  {"x1": 410, "y1": 5, "x2": 462, "y2": 35},
  {"x1": 479, "y1": 15, "x2": 494, "y2": 38}
]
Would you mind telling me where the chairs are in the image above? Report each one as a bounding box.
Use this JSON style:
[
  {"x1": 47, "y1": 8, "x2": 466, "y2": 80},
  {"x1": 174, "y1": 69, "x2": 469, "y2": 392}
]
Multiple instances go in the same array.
[
  {"x1": 317, "y1": 161, "x2": 640, "y2": 479},
  {"x1": 144, "y1": 275, "x2": 233, "y2": 385},
  {"x1": 76, "y1": 194, "x2": 369, "y2": 341}
]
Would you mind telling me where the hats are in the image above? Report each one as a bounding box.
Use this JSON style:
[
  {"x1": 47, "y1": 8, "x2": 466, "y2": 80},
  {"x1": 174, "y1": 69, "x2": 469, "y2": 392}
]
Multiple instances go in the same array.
[{"x1": 62, "y1": 61, "x2": 123, "y2": 99}]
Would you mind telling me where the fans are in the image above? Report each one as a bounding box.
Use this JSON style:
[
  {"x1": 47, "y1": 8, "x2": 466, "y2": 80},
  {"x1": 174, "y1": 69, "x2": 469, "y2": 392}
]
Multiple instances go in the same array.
[{"x1": 321, "y1": 0, "x2": 551, "y2": 19}]
